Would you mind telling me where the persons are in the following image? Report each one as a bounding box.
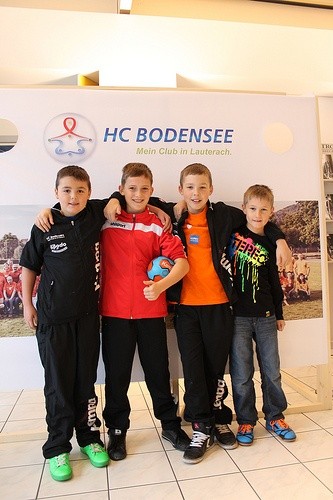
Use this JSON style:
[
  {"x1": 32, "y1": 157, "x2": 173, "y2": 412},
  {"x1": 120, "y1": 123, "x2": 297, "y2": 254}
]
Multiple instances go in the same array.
[
  {"x1": 34, "y1": 163, "x2": 192, "y2": 461},
  {"x1": 3, "y1": 276, "x2": 17, "y2": 317},
  {"x1": 296, "y1": 273, "x2": 311, "y2": 302},
  {"x1": 173, "y1": 184, "x2": 296, "y2": 446},
  {"x1": 294, "y1": 253, "x2": 310, "y2": 277},
  {"x1": 323, "y1": 155, "x2": 333, "y2": 179},
  {"x1": 104, "y1": 163, "x2": 292, "y2": 464},
  {"x1": 0, "y1": 258, "x2": 41, "y2": 310},
  {"x1": 19, "y1": 165, "x2": 173, "y2": 482},
  {"x1": 278, "y1": 254, "x2": 298, "y2": 306}
]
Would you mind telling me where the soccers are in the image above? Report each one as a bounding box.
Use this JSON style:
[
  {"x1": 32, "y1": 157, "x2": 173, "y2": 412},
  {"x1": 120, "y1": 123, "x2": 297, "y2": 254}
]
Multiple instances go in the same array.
[{"x1": 146, "y1": 256, "x2": 176, "y2": 282}]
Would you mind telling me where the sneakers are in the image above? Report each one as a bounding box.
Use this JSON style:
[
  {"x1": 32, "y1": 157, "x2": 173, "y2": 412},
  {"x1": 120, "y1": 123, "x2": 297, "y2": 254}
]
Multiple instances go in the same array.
[
  {"x1": 47, "y1": 443, "x2": 110, "y2": 482},
  {"x1": 106, "y1": 429, "x2": 128, "y2": 461},
  {"x1": 161, "y1": 423, "x2": 237, "y2": 464},
  {"x1": 236, "y1": 418, "x2": 297, "y2": 446}
]
